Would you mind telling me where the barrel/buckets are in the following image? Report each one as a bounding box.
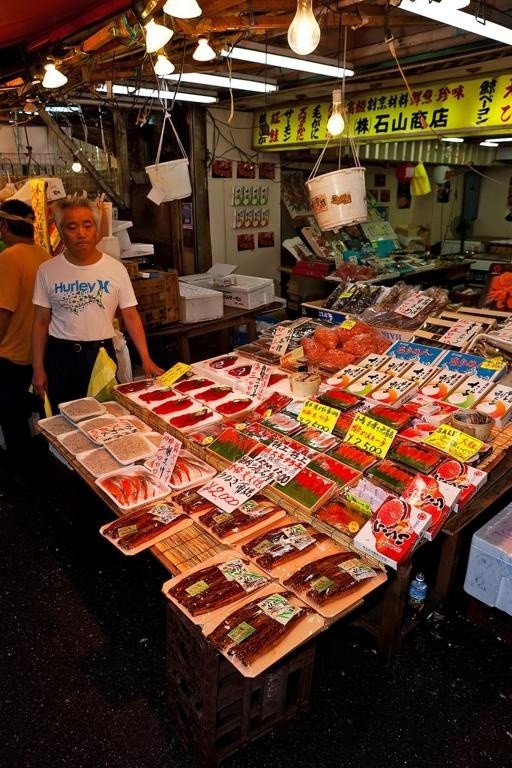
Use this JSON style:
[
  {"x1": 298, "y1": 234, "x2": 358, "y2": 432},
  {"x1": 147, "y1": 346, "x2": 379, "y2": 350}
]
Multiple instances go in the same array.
[
  {"x1": 144, "y1": 157, "x2": 193, "y2": 204},
  {"x1": 304, "y1": 166, "x2": 369, "y2": 232}
]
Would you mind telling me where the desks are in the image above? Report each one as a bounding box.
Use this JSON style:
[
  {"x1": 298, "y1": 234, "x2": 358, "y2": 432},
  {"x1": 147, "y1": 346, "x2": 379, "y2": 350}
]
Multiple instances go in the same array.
[
  {"x1": 127, "y1": 301, "x2": 287, "y2": 364},
  {"x1": 276, "y1": 237, "x2": 512, "y2": 317}
]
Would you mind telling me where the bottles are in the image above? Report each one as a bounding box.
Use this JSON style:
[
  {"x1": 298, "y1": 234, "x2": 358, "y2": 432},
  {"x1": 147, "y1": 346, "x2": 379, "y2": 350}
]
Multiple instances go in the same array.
[{"x1": 409, "y1": 572, "x2": 428, "y2": 612}]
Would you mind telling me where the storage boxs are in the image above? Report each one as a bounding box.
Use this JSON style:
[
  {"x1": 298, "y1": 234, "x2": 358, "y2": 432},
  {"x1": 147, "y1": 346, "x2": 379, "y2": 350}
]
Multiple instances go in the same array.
[
  {"x1": 212, "y1": 274, "x2": 275, "y2": 310},
  {"x1": 463, "y1": 500, "x2": 512, "y2": 618},
  {"x1": 178, "y1": 282, "x2": 224, "y2": 325},
  {"x1": 114, "y1": 269, "x2": 180, "y2": 333}
]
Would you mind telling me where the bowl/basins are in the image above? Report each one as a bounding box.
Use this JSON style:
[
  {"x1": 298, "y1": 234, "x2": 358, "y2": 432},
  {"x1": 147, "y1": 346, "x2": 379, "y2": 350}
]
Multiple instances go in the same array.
[
  {"x1": 449, "y1": 411, "x2": 495, "y2": 443},
  {"x1": 289, "y1": 371, "x2": 321, "y2": 395},
  {"x1": 454, "y1": 286, "x2": 480, "y2": 307}
]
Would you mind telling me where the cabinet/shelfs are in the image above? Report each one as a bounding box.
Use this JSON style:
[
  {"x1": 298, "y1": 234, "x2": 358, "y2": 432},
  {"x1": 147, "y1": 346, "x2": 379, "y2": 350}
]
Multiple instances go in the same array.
[{"x1": 34, "y1": 317, "x2": 512, "y2": 767}]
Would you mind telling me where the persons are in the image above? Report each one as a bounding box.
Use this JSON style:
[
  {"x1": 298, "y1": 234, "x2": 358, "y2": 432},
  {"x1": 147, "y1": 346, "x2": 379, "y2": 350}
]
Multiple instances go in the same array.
[
  {"x1": 31, "y1": 197, "x2": 164, "y2": 416},
  {"x1": 1, "y1": 199, "x2": 56, "y2": 455}
]
